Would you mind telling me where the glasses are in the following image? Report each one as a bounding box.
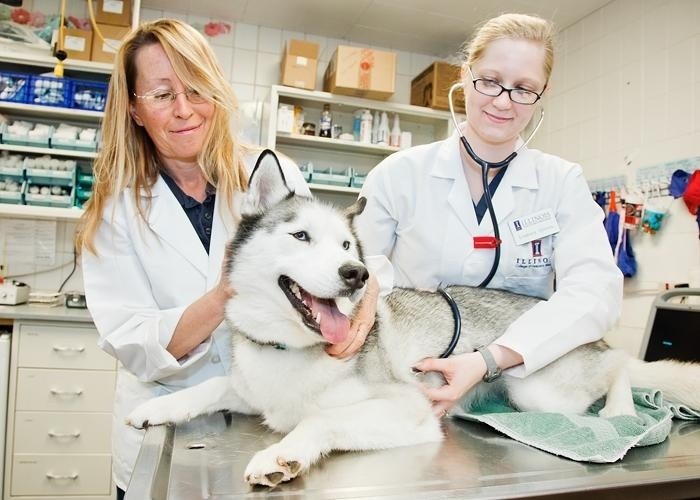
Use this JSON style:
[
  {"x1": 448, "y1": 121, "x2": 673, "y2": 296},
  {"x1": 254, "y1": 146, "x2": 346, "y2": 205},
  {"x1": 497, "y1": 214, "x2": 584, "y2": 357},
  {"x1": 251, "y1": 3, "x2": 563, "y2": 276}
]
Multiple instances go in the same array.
[
  {"x1": 132, "y1": 86, "x2": 208, "y2": 108},
  {"x1": 467, "y1": 65, "x2": 546, "y2": 105}
]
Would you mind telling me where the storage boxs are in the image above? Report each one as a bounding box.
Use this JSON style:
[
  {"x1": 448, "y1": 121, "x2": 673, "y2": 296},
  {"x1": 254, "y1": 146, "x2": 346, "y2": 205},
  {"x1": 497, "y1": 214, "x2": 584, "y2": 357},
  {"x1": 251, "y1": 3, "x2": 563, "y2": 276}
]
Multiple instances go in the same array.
[
  {"x1": 279, "y1": 37, "x2": 320, "y2": 90},
  {"x1": 410, "y1": 61, "x2": 467, "y2": 112},
  {"x1": 96, "y1": 0, "x2": 131, "y2": 26},
  {"x1": 91, "y1": 24, "x2": 130, "y2": 64},
  {"x1": 324, "y1": 45, "x2": 397, "y2": 101},
  {"x1": 56, "y1": 28, "x2": 93, "y2": 62}
]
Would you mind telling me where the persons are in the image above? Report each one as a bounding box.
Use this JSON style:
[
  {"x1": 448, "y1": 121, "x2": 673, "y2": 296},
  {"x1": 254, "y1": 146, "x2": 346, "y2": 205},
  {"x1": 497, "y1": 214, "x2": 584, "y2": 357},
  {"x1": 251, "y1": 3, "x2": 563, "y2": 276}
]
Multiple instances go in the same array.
[
  {"x1": 353, "y1": 14, "x2": 626, "y2": 422},
  {"x1": 74, "y1": 16, "x2": 314, "y2": 500}
]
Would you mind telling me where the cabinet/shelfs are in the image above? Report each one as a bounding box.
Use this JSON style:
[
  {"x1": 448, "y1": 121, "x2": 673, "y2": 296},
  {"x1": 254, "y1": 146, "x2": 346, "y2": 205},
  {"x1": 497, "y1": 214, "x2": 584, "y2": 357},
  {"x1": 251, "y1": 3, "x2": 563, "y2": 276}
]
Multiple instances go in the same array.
[
  {"x1": 0, "y1": 49, "x2": 117, "y2": 223},
  {"x1": 259, "y1": 83, "x2": 467, "y2": 215}
]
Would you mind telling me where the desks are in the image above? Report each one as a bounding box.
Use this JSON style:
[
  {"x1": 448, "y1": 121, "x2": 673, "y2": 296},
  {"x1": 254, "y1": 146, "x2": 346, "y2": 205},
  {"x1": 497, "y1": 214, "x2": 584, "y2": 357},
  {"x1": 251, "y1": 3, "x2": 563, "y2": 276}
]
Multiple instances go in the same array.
[
  {"x1": 1, "y1": 302, "x2": 118, "y2": 500},
  {"x1": 123, "y1": 409, "x2": 700, "y2": 500}
]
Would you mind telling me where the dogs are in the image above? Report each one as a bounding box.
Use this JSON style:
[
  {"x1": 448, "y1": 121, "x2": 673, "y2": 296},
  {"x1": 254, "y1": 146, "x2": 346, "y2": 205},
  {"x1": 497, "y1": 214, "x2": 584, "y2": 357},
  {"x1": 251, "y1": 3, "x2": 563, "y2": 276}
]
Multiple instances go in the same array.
[{"x1": 125, "y1": 148, "x2": 700, "y2": 488}]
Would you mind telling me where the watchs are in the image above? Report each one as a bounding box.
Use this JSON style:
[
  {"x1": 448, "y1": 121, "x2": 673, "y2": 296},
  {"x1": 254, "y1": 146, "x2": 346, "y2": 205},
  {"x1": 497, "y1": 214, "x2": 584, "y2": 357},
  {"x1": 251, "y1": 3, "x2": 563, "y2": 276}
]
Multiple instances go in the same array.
[{"x1": 474, "y1": 343, "x2": 503, "y2": 385}]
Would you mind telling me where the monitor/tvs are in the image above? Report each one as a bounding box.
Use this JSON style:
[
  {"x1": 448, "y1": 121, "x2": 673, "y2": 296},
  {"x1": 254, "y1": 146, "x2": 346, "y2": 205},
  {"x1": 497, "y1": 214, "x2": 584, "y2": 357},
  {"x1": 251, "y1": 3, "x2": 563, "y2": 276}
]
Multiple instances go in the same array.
[{"x1": 636, "y1": 287, "x2": 700, "y2": 362}]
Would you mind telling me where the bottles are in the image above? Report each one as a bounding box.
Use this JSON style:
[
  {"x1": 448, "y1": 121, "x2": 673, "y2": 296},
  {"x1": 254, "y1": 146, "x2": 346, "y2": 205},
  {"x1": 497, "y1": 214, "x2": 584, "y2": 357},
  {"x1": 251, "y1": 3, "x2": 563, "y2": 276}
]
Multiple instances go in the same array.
[
  {"x1": 377, "y1": 112, "x2": 389, "y2": 146},
  {"x1": 373, "y1": 111, "x2": 379, "y2": 144},
  {"x1": 319, "y1": 104, "x2": 332, "y2": 138},
  {"x1": 359, "y1": 109, "x2": 373, "y2": 144},
  {"x1": 391, "y1": 114, "x2": 401, "y2": 147}
]
